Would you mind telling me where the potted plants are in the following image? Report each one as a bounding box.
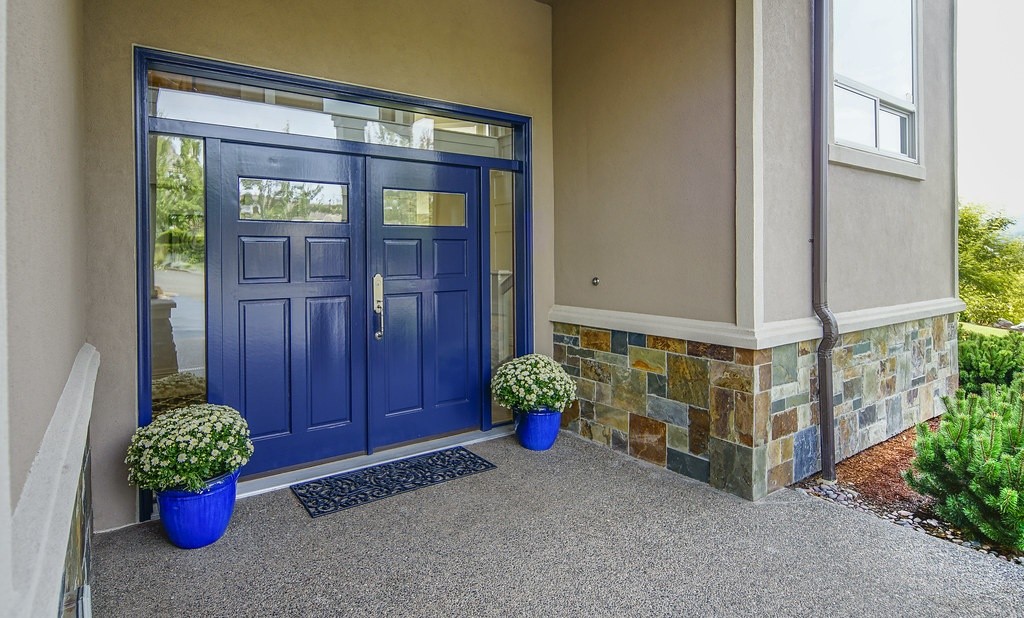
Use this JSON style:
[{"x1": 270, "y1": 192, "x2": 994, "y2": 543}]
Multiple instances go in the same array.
[{"x1": 155, "y1": 469, "x2": 238, "y2": 549}]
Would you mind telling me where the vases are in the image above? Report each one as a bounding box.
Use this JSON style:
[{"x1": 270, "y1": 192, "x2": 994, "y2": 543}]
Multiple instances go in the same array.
[{"x1": 512, "y1": 407, "x2": 562, "y2": 452}]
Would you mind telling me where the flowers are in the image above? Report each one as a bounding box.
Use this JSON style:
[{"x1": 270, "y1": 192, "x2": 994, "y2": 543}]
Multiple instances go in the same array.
[
  {"x1": 122, "y1": 402, "x2": 254, "y2": 491},
  {"x1": 490, "y1": 354, "x2": 576, "y2": 412}
]
